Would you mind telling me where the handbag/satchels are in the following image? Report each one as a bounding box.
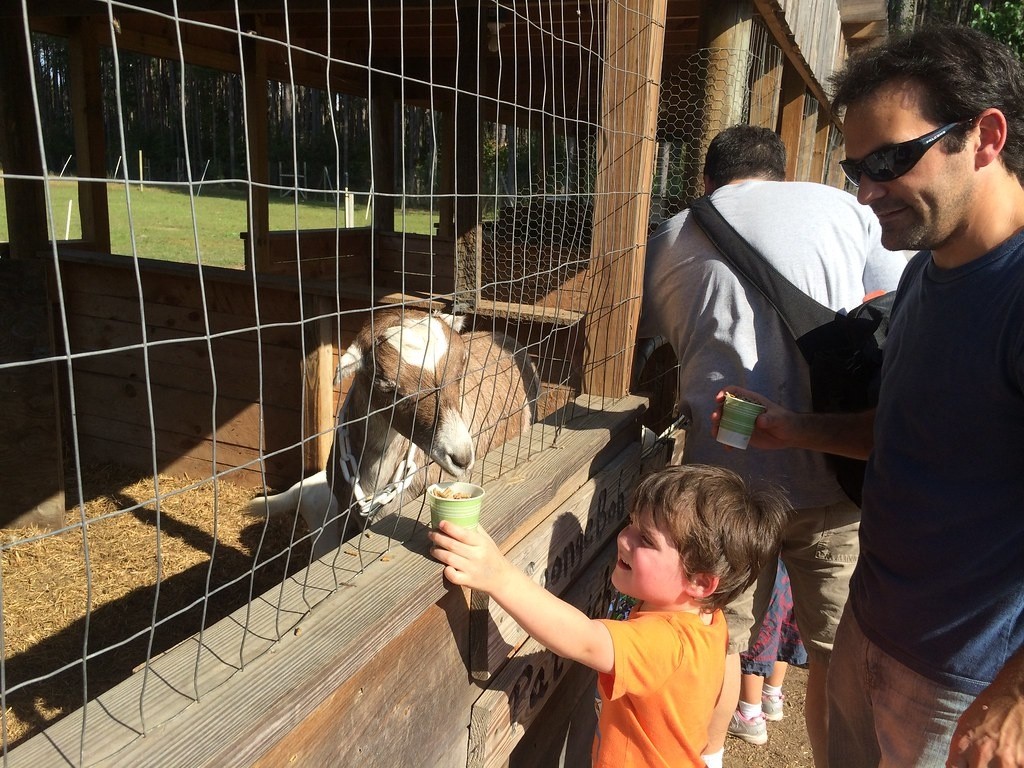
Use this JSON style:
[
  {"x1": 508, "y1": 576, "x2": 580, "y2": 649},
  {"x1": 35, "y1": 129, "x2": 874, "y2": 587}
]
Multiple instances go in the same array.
[{"x1": 794, "y1": 289, "x2": 896, "y2": 508}]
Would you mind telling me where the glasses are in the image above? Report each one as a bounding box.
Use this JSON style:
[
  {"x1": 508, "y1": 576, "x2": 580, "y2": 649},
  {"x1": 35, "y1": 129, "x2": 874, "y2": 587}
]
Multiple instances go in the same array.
[{"x1": 838, "y1": 119, "x2": 972, "y2": 187}]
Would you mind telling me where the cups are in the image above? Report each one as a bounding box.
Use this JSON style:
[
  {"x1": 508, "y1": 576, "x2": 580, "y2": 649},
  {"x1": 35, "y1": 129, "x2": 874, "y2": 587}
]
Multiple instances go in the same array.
[
  {"x1": 427, "y1": 481, "x2": 485, "y2": 548},
  {"x1": 717, "y1": 394, "x2": 765, "y2": 451}
]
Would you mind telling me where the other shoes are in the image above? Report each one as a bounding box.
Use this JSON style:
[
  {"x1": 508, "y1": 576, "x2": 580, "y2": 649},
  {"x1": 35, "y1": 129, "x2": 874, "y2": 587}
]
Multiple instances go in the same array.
[
  {"x1": 727, "y1": 709, "x2": 768, "y2": 745},
  {"x1": 700, "y1": 746, "x2": 724, "y2": 768},
  {"x1": 761, "y1": 690, "x2": 784, "y2": 721}
]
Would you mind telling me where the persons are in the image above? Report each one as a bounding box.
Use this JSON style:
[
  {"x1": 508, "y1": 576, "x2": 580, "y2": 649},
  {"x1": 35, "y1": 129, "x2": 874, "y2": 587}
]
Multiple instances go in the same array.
[
  {"x1": 428, "y1": 464, "x2": 798, "y2": 768},
  {"x1": 634, "y1": 22, "x2": 1024, "y2": 768}
]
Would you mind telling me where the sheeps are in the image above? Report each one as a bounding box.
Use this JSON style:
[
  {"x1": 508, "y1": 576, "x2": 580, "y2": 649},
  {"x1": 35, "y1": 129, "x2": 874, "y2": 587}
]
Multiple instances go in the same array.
[{"x1": 247, "y1": 307, "x2": 542, "y2": 562}]
